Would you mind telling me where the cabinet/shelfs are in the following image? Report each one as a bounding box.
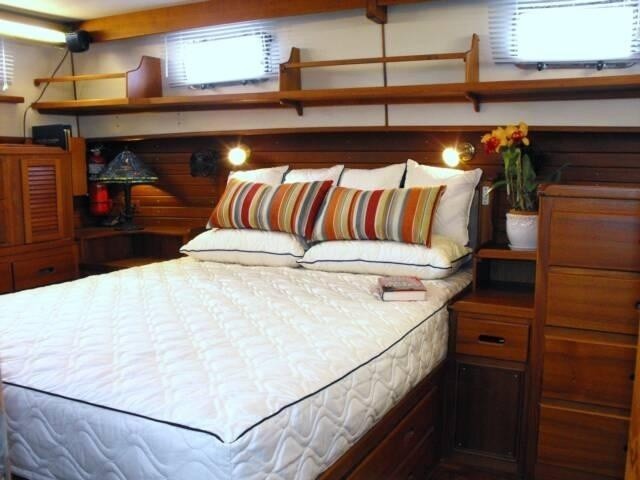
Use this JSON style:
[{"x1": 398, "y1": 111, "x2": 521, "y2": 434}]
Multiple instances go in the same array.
[
  {"x1": 1, "y1": 136, "x2": 86, "y2": 293},
  {"x1": 526, "y1": 184, "x2": 640, "y2": 480}
]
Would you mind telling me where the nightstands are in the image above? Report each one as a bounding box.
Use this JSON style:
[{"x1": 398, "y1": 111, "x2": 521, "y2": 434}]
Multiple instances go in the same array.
[
  {"x1": 440, "y1": 248, "x2": 537, "y2": 477},
  {"x1": 77, "y1": 226, "x2": 200, "y2": 270}
]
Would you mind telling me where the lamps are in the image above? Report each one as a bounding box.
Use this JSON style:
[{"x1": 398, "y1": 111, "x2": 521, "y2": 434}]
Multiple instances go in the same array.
[
  {"x1": 441, "y1": 142, "x2": 476, "y2": 168},
  {"x1": 225, "y1": 144, "x2": 252, "y2": 164},
  {"x1": 95, "y1": 145, "x2": 162, "y2": 230}
]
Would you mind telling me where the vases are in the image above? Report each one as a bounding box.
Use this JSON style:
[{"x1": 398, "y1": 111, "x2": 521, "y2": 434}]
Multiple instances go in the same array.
[{"x1": 504, "y1": 209, "x2": 540, "y2": 254}]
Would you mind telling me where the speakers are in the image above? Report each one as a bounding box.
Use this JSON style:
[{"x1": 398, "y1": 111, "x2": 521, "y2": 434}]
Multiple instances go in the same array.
[{"x1": 64, "y1": 31, "x2": 90, "y2": 53}]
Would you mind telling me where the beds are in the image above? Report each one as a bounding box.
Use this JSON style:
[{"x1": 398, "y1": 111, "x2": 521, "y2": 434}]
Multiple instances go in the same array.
[{"x1": 2, "y1": 168, "x2": 496, "y2": 480}]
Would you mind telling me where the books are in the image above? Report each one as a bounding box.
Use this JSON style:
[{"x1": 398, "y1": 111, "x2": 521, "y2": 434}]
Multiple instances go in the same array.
[{"x1": 377, "y1": 274, "x2": 427, "y2": 301}]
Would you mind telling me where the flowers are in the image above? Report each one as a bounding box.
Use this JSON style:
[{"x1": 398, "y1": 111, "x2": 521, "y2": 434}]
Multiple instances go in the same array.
[{"x1": 480, "y1": 121, "x2": 572, "y2": 213}]
